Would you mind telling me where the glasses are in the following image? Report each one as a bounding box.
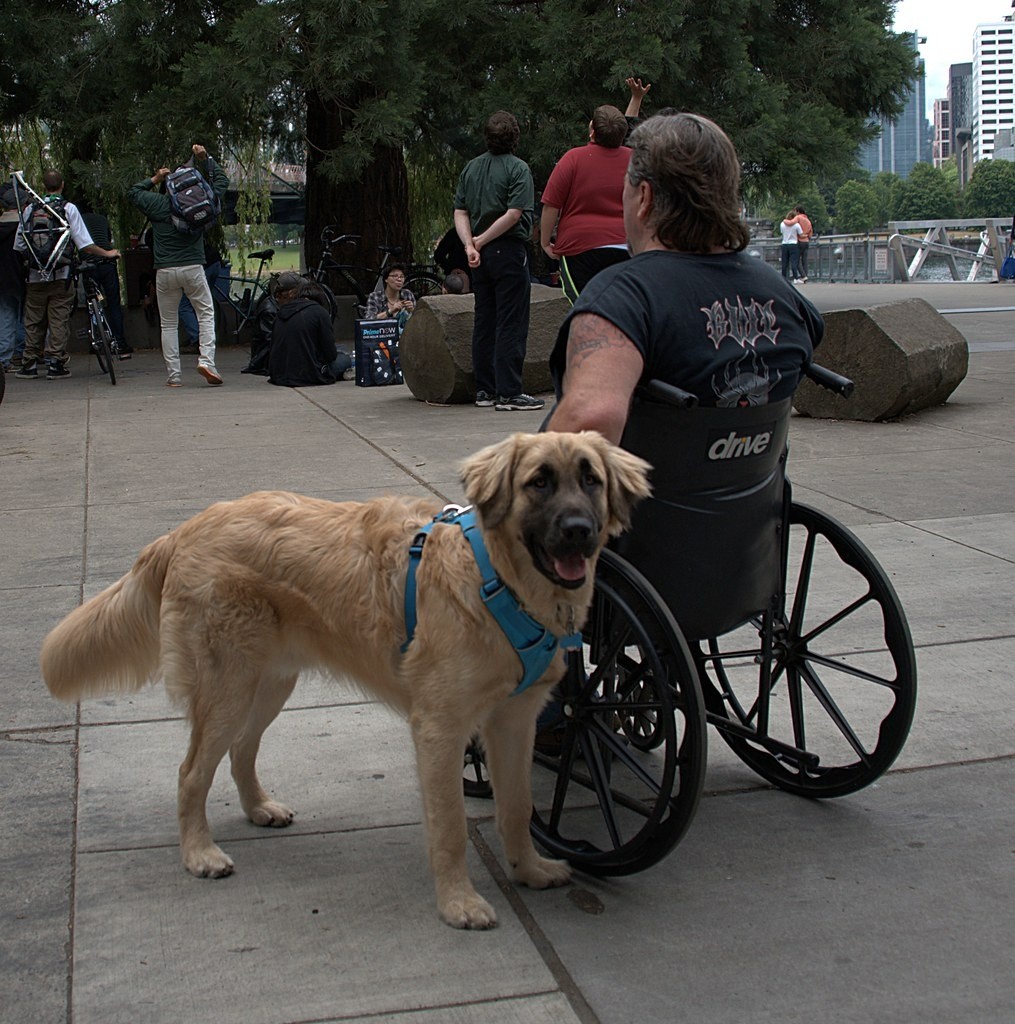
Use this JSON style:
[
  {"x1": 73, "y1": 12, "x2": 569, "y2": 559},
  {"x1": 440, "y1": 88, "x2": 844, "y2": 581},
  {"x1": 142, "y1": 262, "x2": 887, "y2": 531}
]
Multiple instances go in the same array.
[{"x1": 388, "y1": 273, "x2": 404, "y2": 277}]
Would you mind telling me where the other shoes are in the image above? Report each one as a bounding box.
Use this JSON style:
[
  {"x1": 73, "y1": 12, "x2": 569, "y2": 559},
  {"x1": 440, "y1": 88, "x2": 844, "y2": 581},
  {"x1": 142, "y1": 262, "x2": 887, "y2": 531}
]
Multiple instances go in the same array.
[
  {"x1": 534, "y1": 698, "x2": 624, "y2": 761},
  {"x1": 65, "y1": 356, "x2": 70, "y2": 364},
  {"x1": 4, "y1": 363, "x2": 22, "y2": 373},
  {"x1": 196, "y1": 362, "x2": 224, "y2": 385},
  {"x1": 166, "y1": 372, "x2": 183, "y2": 387}
]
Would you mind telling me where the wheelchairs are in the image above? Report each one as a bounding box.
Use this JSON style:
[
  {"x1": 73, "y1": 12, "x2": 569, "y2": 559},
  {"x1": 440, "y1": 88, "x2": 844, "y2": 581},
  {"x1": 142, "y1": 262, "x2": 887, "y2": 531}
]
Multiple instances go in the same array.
[{"x1": 459, "y1": 365, "x2": 918, "y2": 879}]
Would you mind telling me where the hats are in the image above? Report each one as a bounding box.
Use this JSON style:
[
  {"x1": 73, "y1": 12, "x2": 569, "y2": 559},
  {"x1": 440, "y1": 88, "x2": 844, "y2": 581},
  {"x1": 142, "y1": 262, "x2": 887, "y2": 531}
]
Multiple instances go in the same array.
[{"x1": 277, "y1": 271, "x2": 308, "y2": 292}]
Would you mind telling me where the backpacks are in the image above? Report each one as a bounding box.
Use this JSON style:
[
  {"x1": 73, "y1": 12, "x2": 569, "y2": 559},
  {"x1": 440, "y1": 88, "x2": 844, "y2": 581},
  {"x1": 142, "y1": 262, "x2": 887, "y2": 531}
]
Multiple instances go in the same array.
[
  {"x1": 163, "y1": 151, "x2": 220, "y2": 236},
  {"x1": 22, "y1": 199, "x2": 72, "y2": 270}
]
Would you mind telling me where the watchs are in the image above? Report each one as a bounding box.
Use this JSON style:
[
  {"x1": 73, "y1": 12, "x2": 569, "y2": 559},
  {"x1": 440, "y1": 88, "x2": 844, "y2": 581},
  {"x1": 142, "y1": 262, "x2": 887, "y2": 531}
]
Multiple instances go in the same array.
[{"x1": 386, "y1": 308, "x2": 393, "y2": 317}]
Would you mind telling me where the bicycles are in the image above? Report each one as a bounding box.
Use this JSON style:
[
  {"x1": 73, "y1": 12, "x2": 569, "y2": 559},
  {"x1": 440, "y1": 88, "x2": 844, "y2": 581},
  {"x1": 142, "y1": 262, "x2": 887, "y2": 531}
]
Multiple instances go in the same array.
[
  {"x1": 198, "y1": 248, "x2": 338, "y2": 342},
  {"x1": 301, "y1": 222, "x2": 452, "y2": 318},
  {"x1": 68, "y1": 256, "x2": 117, "y2": 387}
]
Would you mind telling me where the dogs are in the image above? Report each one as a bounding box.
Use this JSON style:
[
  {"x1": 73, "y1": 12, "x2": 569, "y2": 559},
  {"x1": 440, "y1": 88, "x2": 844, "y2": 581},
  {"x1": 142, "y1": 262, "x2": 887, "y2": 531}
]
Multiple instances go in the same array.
[{"x1": 37, "y1": 430, "x2": 654, "y2": 930}]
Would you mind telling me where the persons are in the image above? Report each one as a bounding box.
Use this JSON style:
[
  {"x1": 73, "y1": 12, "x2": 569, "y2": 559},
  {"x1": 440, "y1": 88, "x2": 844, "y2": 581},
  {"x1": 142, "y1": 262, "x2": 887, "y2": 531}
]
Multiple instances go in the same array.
[
  {"x1": 783, "y1": 206, "x2": 812, "y2": 284},
  {"x1": 541, "y1": 105, "x2": 632, "y2": 309},
  {"x1": 779, "y1": 211, "x2": 808, "y2": 284},
  {"x1": 267, "y1": 283, "x2": 352, "y2": 389},
  {"x1": 127, "y1": 144, "x2": 230, "y2": 386},
  {"x1": 365, "y1": 263, "x2": 416, "y2": 338},
  {"x1": 455, "y1": 110, "x2": 546, "y2": 411},
  {"x1": 538, "y1": 111, "x2": 825, "y2": 759},
  {"x1": 443, "y1": 269, "x2": 469, "y2": 294},
  {"x1": 71, "y1": 195, "x2": 135, "y2": 359},
  {"x1": 12, "y1": 170, "x2": 122, "y2": 380},
  {"x1": 146, "y1": 225, "x2": 222, "y2": 353},
  {"x1": 623, "y1": 77, "x2": 681, "y2": 148},
  {"x1": 983, "y1": 212, "x2": 1015, "y2": 279},
  {"x1": 242, "y1": 271, "x2": 308, "y2": 376},
  {"x1": 0, "y1": 183, "x2": 34, "y2": 373}
]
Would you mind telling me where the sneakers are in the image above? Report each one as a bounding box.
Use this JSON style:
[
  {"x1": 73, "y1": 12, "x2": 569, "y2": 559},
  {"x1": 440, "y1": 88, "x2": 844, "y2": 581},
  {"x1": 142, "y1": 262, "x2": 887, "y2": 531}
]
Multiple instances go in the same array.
[
  {"x1": 474, "y1": 390, "x2": 498, "y2": 407},
  {"x1": 46, "y1": 366, "x2": 71, "y2": 380},
  {"x1": 495, "y1": 394, "x2": 546, "y2": 411},
  {"x1": 15, "y1": 368, "x2": 38, "y2": 379}
]
